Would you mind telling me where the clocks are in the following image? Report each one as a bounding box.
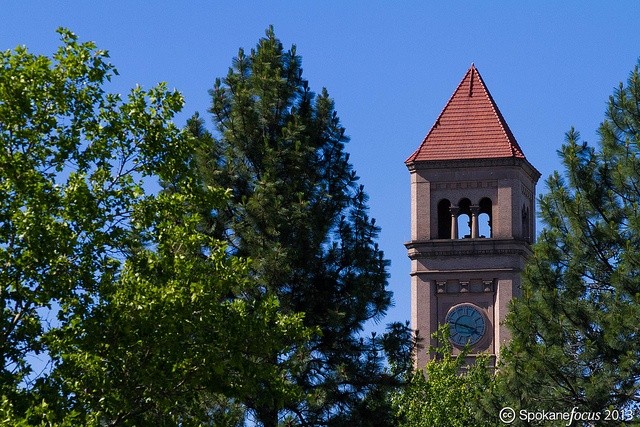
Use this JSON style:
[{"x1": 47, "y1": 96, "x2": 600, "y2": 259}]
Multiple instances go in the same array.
[{"x1": 444, "y1": 303, "x2": 487, "y2": 349}]
